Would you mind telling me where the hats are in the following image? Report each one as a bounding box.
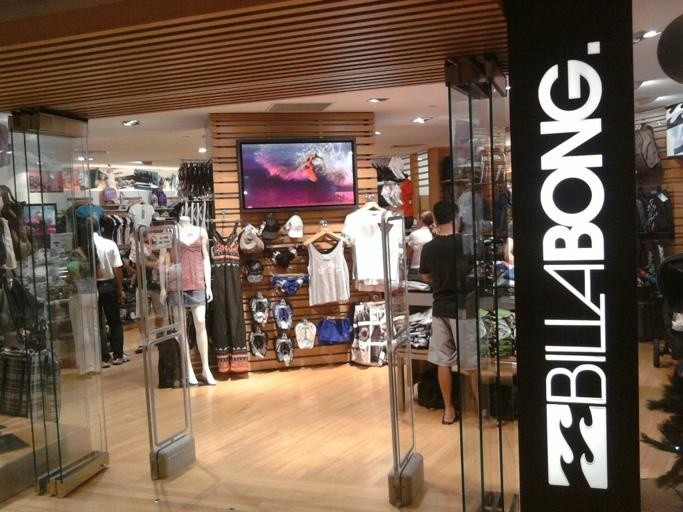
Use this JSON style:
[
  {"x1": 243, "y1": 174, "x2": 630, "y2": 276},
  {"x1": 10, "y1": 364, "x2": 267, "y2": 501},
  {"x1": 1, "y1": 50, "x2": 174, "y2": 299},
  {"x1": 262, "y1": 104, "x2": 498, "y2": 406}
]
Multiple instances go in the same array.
[
  {"x1": 284, "y1": 215, "x2": 304, "y2": 238},
  {"x1": 240, "y1": 260, "x2": 264, "y2": 283},
  {"x1": 238, "y1": 225, "x2": 264, "y2": 255},
  {"x1": 262, "y1": 216, "x2": 281, "y2": 240}
]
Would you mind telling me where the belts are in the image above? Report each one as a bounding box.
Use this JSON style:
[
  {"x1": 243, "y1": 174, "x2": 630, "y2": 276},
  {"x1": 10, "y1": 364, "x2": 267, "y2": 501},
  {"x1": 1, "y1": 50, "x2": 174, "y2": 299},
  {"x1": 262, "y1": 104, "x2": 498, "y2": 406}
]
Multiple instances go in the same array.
[{"x1": 96, "y1": 279, "x2": 114, "y2": 284}]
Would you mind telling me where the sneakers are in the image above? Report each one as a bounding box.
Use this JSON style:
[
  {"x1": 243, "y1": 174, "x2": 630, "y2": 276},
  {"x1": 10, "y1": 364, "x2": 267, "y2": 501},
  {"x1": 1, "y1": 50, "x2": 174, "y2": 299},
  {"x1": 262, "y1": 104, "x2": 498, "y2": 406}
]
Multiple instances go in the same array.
[
  {"x1": 112, "y1": 354, "x2": 130, "y2": 365},
  {"x1": 135, "y1": 344, "x2": 148, "y2": 354},
  {"x1": 102, "y1": 360, "x2": 110, "y2": 368}
]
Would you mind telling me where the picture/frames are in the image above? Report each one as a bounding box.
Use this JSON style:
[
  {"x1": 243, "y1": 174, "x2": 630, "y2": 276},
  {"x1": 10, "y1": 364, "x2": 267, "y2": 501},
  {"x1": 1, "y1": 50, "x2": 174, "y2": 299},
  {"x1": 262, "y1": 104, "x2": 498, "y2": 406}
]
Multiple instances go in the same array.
[
  {"x1": 28, "y1": 170, "x2": 63, "y2": 192},
  {"x1": 21, "y1": 203, "x2": 58, "y2": 239}
]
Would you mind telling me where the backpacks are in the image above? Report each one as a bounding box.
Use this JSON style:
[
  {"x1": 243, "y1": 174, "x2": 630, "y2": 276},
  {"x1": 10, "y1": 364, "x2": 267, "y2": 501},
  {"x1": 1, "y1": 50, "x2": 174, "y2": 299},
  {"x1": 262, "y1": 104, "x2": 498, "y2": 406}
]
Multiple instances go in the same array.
[
  {"x1": 635, "y1": 186, "x2": 651, "y2": 234},
  {"x1": 0, "y1": 123, "x2": 14, "y2": 169},
  {"x1": 633, "y1": 122, "x2": 661, "y2": 175},
  {"x1": 646, "y1": 243, "x2": 667, "y2": 280},
  {"x1": 645, "y1": 184, "x2": 675, "y2": 239}
]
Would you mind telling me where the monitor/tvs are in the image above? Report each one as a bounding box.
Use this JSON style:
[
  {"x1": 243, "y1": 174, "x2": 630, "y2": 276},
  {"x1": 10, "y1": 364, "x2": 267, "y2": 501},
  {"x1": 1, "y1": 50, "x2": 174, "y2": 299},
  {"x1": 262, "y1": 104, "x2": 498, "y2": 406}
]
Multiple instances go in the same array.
[{"x1": 235, "y1": 135, "x2": 358, "y2": 215}]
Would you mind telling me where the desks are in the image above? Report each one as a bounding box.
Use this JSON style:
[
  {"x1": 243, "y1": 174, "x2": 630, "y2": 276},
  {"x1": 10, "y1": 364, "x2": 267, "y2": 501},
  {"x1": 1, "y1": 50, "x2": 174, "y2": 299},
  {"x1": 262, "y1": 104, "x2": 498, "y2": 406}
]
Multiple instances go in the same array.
[{"x1": 394, "y1": 346, "x2": 517, "y2": 414}]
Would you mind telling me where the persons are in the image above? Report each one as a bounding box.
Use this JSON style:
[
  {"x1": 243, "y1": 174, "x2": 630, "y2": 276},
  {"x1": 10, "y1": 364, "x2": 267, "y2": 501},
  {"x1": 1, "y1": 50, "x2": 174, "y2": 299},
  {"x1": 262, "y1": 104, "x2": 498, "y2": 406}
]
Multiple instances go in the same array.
[
  {"x1": 64, "y1": 216, "x2": 104, "y2": 378},
  {"x1": 406, "y1": 210, "x2": 437, "y2": 281},
  {"x1": 503, "y1": 222, "x2": 514, "y2": 266},
  {"x1": 159, "y1": 216, "x2": 217, "y2": 386},
  {"x1": 130, "y1": 219, "x2": 167, "y2": 355},
  {"x1": 419, "y1": 199, "x2": 506, "y2": 429},
  {"x1": 86, "y1": 216, "x2": 131, "y2": 369},
  {"x1": 128, "y1": 224, "x2": 161, "y2": 290}
]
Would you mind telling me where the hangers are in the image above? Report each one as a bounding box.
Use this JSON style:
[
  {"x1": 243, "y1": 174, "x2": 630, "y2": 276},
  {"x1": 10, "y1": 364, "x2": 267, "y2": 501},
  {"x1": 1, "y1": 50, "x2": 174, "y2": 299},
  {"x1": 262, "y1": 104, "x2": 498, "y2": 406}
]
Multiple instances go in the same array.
[
  {"x1": 101, "y1": 198, "x2": 154, "y2": 224},
  {"x1": 302, "y1": 219, "x2": 350, "y2": 247},
  {"x1": 344, "y1": 194, "x2": 395, "y2": 217}
]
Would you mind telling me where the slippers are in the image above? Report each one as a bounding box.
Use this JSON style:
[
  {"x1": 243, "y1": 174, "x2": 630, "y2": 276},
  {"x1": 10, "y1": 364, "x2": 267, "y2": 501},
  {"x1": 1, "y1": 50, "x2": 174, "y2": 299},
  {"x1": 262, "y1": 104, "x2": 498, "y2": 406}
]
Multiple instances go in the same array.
[
  {"x1": 249, "y1": 295, "x2": 294, "y2": 362},
  {"x1": 295, "y1": 322, "x2": 317, "y2": 350},
  {"x1": 442, "y1": 409, "x2": 461, "y2": 424}
]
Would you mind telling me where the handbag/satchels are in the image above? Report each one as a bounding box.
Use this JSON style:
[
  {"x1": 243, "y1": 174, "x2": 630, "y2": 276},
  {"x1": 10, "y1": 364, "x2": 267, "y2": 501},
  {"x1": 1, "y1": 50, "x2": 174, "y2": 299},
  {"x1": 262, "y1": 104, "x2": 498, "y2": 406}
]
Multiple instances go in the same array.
[
  {"x1": 346, "y1": 300, "x2": 390, "y2": 367},
  {"x1": 0, "y1": 182, "x2": 34, "y2": 272},
  {"x1": 0, "y1": 272, "x2": 44, "y2": 336},
  {"x1": 102, "y1": 187, "x2": 117, "y2": 201},
  {"x1": 417, "y1": 368, "x2": 460, "y2": 410},
  {"x1": 153, "y1": 189, "x2": 167, "y2": 208},
  {"x1": 0, "y1": 343, "x2": 60, "y2": 424}
]
